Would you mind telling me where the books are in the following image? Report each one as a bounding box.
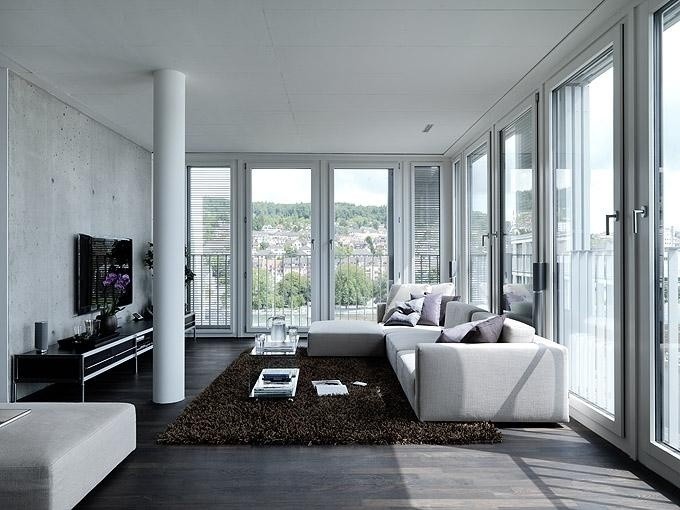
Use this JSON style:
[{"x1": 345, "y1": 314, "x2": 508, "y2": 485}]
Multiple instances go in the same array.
[
  {"x1": 0, "y1": 409, "x2": 32, "y2": 426},
  {"x1": 310, "y1": 379, "x2": 349, "y2": 397},
  {"x1": 254, "y1": 373, "x2": 296, "y2": 396}
]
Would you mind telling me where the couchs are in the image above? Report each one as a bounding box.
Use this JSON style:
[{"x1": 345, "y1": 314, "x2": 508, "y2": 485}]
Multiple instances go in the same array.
[{"x1": 375, "y1": 299, "x2": 571, "y2": 425}]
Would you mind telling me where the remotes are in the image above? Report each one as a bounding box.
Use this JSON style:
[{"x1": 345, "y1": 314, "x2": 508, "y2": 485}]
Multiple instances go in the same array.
[{"x1": 325, "y1": 383, "x2": 337, "y2": 385}]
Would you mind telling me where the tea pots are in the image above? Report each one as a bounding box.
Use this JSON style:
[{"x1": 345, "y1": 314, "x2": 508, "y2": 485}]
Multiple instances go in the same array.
[{"x1": 266, "y1": 315, "x2": 287, "y2": 342}]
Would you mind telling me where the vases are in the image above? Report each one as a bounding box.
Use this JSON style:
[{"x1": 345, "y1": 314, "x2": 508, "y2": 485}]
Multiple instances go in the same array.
[{"x1": 94, "y1": 315, "x2": 117, "y2": 336}]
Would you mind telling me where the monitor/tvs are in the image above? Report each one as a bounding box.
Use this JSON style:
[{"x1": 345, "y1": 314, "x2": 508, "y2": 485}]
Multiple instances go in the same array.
[{"x1": 77, "y1": 232, "x2": 134, "y2": 316}]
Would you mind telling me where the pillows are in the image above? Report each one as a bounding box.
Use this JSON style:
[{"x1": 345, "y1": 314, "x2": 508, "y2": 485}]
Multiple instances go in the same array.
[
  {"x1": 381, "y1": 281, "x2": 461, "y2": 327},
  {"x1": 435, "y1": 313, "x2": 507, "y2": 343}
]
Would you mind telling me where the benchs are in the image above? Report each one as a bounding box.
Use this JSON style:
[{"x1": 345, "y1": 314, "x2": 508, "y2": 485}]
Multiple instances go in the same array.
[
  {"x1": 307, "y1": 319, "x2": 384, "y2": 358},
  {"x1": 0, "y1": 402, "x2": 136, "y2": 510}
]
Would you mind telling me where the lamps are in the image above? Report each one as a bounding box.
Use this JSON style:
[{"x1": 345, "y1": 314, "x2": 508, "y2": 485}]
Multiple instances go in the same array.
[{"x1": 533, "y1": 261, "x2": 546, "y2": 294}]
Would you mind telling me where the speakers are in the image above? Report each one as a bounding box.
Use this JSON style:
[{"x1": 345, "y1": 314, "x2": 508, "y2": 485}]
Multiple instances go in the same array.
[{"x1": 34, "y1": 320, "x2": 49, "y2": 353}]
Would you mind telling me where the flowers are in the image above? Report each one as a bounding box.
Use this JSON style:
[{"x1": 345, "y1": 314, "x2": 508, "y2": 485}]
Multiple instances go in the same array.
[{"x1": 99, "y1": 271, "x2": 130, "y2": 315}]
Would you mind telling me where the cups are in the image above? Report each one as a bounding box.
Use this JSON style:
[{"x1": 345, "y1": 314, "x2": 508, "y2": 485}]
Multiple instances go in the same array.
[
  {"x1": 255, "y1": 333, "x2": 265, "y2": 351},
  {"x1": 73, "y1": 324, "x2": 82, "y2": 344},
  {"x1": 288, "y1": 326, "x2": 298, "y2": 340}
]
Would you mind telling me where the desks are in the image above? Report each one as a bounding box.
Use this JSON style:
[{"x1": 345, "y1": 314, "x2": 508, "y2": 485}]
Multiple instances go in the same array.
[{"x1": 11, "y1": 310, "x2": 197, "y2": 402}]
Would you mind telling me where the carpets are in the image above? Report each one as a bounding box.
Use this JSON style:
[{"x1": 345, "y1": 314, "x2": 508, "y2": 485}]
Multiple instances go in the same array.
[{"x1": 154, "y1": 346, "x2": 504, "y2": 448}]
